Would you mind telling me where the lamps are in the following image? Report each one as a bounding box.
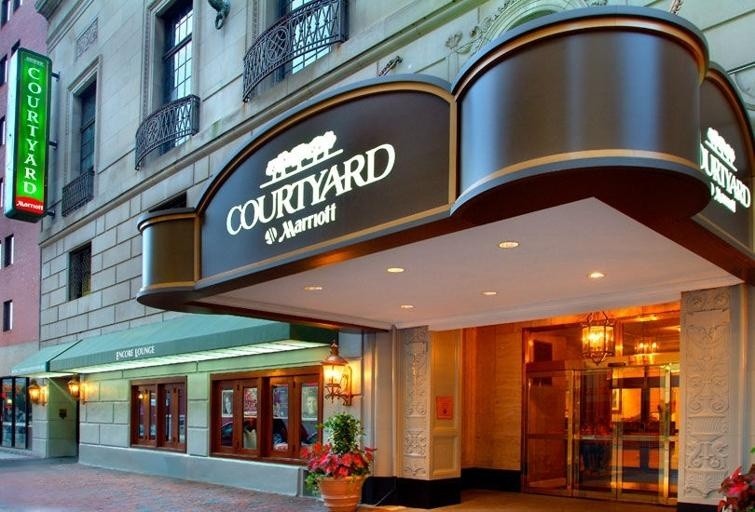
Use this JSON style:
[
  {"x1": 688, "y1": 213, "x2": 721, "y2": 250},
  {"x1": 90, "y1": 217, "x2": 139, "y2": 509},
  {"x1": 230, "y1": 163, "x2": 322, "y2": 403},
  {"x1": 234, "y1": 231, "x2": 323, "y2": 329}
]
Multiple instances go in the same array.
[
  {"x1": 67, "y1": 374, "x2": 86, "y2": 405},
  {"x1": 28, "y1": 382, "x2": 46, "y2": 407},
  {"x1": 320, "y1": 339, "x2": 353, "y2": 406},
  {"x1": 581, "y1": 308, "x2": 615, "y2": 364}
]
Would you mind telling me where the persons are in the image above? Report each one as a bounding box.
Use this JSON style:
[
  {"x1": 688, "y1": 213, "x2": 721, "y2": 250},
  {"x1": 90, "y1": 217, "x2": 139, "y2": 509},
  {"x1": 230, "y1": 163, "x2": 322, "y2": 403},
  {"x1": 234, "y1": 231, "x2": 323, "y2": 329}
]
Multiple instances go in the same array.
[
  {"x1": 223, "y1": 393, "x2": 233, "y2": 416},
  {"x1": 302, "y1": 390, "x2": 318, "y2": 420},
  {"x1": 272, "y1": 389, "x2": 282, "y2": 418},
  {"x1": 244, "y1": 392, "x2": 257, "y2": 414}
]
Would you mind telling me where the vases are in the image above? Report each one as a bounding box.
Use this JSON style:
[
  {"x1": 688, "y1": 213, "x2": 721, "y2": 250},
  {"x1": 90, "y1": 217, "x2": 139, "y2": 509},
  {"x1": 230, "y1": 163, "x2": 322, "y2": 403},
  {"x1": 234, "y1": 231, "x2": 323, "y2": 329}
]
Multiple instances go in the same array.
[{"x1": 317, "y1": 475, "x2": 364, "y2": 511}]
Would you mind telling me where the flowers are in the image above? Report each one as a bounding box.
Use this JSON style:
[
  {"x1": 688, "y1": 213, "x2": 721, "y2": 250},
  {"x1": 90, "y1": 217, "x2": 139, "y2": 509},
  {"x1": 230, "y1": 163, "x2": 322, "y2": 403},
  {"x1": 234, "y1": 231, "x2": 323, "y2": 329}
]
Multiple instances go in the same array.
[
  {"x1": 298, "y1": 410, "x2": 379, "y2": 505},
  {"x1": 716, "y1": 447, "x2": 755, "y2": 512}
]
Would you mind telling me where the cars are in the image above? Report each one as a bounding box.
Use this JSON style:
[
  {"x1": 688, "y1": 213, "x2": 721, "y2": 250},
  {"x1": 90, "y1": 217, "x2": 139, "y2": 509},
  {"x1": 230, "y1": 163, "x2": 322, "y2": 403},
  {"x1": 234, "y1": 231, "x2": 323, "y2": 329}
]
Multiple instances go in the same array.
[{"x1": 221, "y1": 416, "x2": 317, "y2": 448}]
